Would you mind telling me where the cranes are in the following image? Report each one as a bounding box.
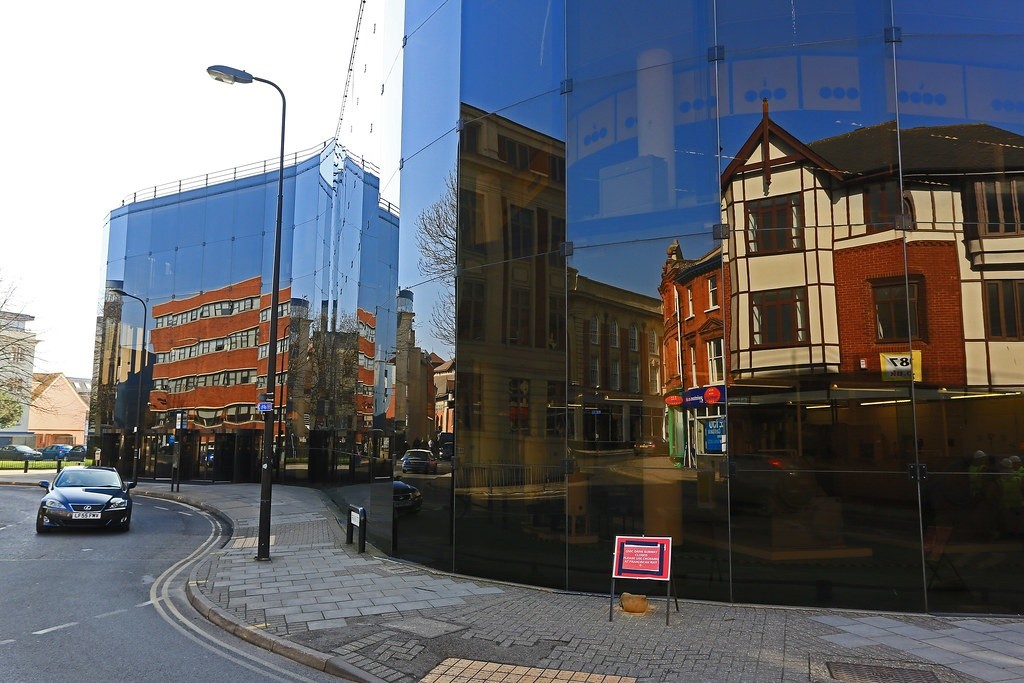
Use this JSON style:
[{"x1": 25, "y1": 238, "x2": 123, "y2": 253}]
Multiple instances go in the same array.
[{"x1": 335, "y1": 0, "x2": 367, "y2": 140}]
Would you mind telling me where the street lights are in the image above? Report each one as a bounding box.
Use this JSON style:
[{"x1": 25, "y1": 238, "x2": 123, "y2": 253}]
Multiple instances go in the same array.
[
  {"x1": 208, "y1": 64, "x2": 287, "y2": 562},
  {"x1": 109, "y1": 288, "x2": 148, "y2": 484}
]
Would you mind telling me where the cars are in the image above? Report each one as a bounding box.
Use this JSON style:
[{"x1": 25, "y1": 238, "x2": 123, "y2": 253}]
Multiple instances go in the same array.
[
  {"x1": 401, "y1": 449, "x2": 437, "y2": 474},
  {"x1": 393, "y1": 476, "x2": 422, "y2": 519},
  {"x1": 439, "y1": 442, "x2": 454, "y2": 461},
  {"x1": 42, "y1": 444, "x2": 73, "y2": 461},
  {"x1": 36, "y1": 465, "x2": 138, "y2": 533},
  {"x1": 0, "y1": 445, "x2": 43, "y2": 461},
  {"x1": 64, "y1": 445, "x2": 88, "y2": 462}
]
